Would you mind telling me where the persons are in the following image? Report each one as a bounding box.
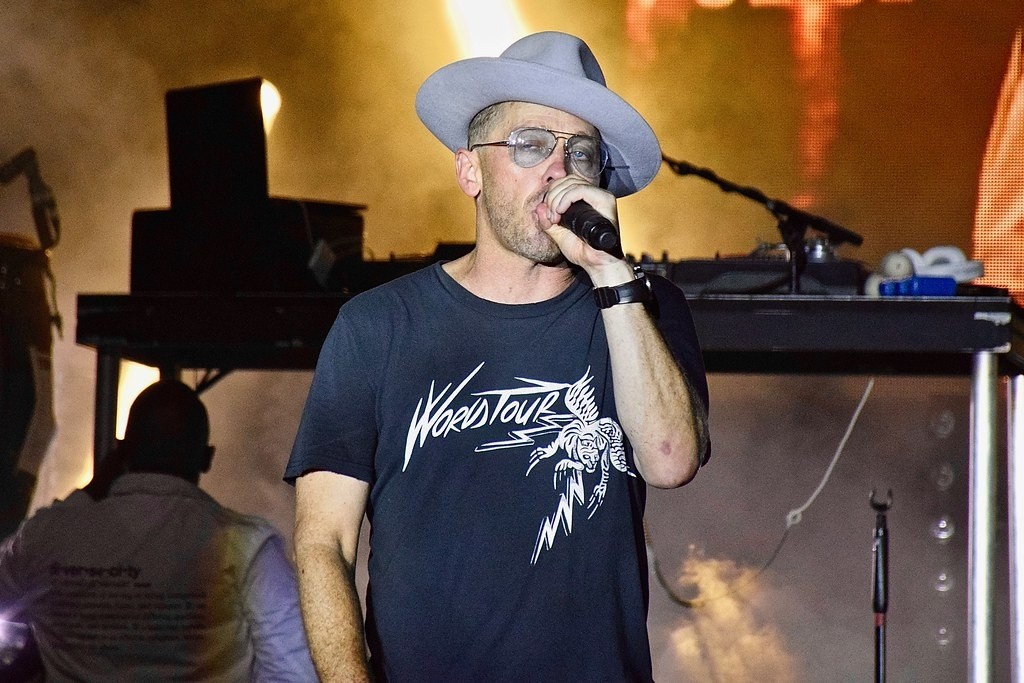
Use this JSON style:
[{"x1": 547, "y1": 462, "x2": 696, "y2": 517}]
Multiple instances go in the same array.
[
  {"x1": 281, "y1": 29, "x2": 709, "y2": 683},
  {"x1": 0, "y1": 379, "x2": 322, "y2": 683}
]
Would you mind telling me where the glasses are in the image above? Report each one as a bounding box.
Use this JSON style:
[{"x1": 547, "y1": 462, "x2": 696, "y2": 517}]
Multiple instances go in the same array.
[{"x1": 468, "y1": 126, "x2": 609, "y2": 179}]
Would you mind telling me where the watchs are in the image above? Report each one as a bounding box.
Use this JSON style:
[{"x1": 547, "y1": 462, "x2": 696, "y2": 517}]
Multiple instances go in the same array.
[{"x1": 593, "y1": 266, "x2": 650, "y2": 310}]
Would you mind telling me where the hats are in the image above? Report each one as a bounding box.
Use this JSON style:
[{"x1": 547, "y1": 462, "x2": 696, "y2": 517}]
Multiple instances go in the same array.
[{"x1": 414, "y1": 32, "x2": 663, "y2": 199}]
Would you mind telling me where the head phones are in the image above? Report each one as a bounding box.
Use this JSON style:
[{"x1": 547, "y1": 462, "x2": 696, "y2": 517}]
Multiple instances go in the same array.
[{"x1": 864, "y1": 245, "x2": 985, "y2": 297}]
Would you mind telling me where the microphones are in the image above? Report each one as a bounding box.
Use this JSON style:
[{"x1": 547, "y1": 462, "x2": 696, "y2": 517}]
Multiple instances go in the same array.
[
  {"x1": 543, "y1": 191, "x2": 619, "y2": 252},
  {"x1": 25, "y1": 154, "x2": 61, "y2": 249}
]
